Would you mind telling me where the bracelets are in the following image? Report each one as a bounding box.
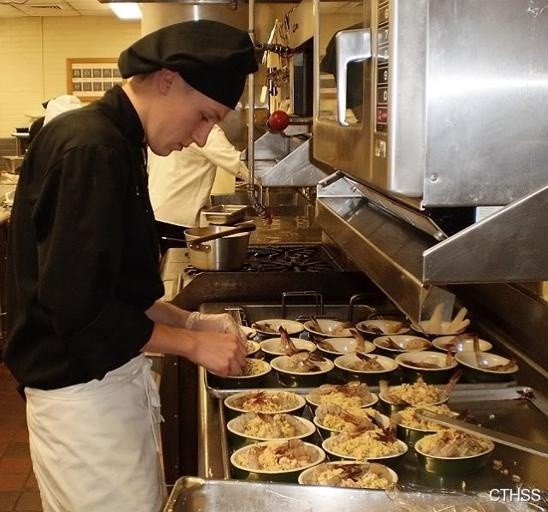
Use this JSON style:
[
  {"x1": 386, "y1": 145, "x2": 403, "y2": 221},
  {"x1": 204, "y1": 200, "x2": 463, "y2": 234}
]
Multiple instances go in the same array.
[{"x1": 183, "y1": 310, "x2": 200, "y2": 330}]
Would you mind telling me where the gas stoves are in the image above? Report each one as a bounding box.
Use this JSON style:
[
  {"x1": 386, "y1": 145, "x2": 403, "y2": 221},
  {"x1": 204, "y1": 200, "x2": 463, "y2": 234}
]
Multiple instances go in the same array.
[{"x1": 241, "y1": 242, "x2": 345, "y2": 274}]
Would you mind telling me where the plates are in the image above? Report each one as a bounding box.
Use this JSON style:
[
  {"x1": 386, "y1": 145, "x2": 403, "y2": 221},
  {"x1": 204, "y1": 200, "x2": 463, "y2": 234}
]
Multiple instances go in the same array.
[
  {"x1": 4, "y1": 188, "x2": 15, "y2": 205},
  {"x1": 10, "y1": 132, "x2": 30, "y2": 139},
  {"x1": 202, "y1": 318, "x2": 522, "y2": 378}
]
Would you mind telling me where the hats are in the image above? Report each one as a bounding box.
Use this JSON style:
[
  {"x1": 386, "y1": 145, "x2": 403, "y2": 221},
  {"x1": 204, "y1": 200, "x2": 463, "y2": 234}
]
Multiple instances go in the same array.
[{"x1": 118, "y1": 20, "x2": 260, "y2": 109}]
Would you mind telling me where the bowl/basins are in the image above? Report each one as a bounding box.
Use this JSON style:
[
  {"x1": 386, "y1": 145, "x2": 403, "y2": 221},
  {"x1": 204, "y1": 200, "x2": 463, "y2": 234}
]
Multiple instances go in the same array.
[
  {"x1": 182, "y1": 226, "x2": 251, "y2": 270},
  {"x1": 223, "y1": 379, "x2": 494, "y2": 492}
]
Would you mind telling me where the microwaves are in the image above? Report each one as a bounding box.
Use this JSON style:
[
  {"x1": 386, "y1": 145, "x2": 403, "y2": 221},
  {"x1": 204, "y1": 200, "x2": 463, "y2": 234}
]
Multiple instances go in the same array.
[{"x1": 311, "y1": 1, "x2": 548, "y2": 212}]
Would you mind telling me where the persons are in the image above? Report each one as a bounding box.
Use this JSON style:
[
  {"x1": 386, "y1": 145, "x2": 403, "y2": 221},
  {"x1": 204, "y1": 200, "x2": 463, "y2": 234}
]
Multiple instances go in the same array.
[
  {"x1": 2, "y1": 19, "x2": 259, "y2": 512},
  {"x1": 146, "y1": 122, "x2": 263, "y2": 257},
  {"x1": 42, "y1": 95, "x2": 83, "y2": 129},
  {"x1": 28, "y1": 96, "x2": 53, "y2": 144}
]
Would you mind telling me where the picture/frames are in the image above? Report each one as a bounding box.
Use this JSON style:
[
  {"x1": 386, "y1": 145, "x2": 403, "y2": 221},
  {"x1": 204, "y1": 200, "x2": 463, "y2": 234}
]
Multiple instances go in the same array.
[{"x1": 65, "y1": 57, "x2": 121, "y2": 102}]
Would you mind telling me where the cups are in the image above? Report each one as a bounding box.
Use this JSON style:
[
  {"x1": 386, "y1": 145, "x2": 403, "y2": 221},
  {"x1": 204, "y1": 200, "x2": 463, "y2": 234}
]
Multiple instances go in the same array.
[{"x1": 15, "y1": 127, "x2": 29, "y2": 139}]
